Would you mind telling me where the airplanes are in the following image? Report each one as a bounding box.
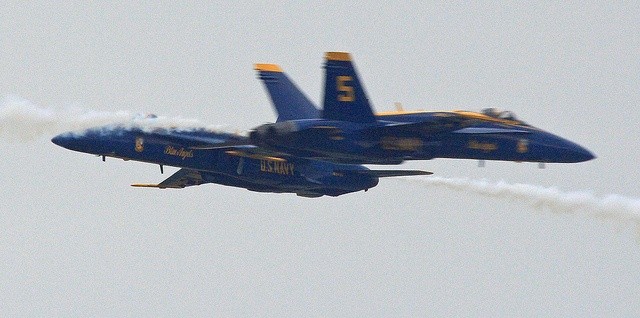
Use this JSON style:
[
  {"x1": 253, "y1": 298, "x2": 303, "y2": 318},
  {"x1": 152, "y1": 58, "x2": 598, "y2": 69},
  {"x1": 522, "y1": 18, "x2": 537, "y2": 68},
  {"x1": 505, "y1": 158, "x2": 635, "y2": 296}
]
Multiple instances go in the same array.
[
  {"x1": 249, "y1": 51, "x2": 596, "y2": 165},
  {"x1": 50, "y1": 117, "x2": 435, "y2": 198}
]
